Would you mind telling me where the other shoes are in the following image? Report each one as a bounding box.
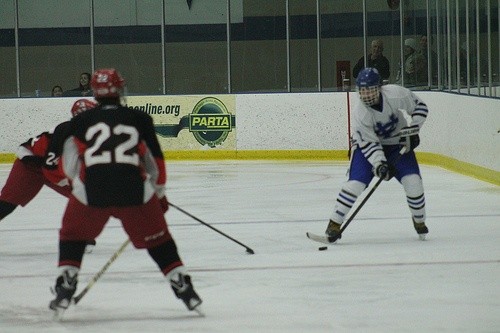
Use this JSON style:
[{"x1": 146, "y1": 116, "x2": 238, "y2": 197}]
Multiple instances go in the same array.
[{"x1": 85, "y1": 239, "x2": 96, "y2": 254}]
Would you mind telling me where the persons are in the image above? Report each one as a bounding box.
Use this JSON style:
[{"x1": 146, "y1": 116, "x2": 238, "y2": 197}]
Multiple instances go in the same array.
[
  {"x1": 49, "y1": 69, "x2": 205, "y2": 320},
  {"x1": 73, "y1": 73, "x2": 91, "y2": 92},
  {"x1": 0, "y1": 99, "x2": 97, "y2": 255},
  {"x1": 52, "y1": 85, "x2": 63, "y2": 97},
  {"x1": 396, "y1": 34, "x2": 437, "y2": 87},
  {"x1": 325, "y1": 67, "x2": 429, "y2": 241},
  {"x1": 353, "y1": 39, "x2": 390, "y2": 80}
]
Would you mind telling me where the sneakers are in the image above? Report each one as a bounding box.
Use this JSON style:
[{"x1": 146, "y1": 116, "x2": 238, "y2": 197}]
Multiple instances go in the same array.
[
  {"x1": 324, "y1": 219, "x2": 343, "y2": 243},
  {"x1": 168, "y1": 271, "x2": 206, "y2": 317},
  {"x1": 49, "y1": 270, "x2": 79, "y2": 322},
  {"x1": 412, "y1": 217, "x2": 430, "y2": 241}
]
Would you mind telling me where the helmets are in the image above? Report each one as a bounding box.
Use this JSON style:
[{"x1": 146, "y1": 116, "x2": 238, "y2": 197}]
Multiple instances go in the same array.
[
  {"x1": 71, "y1": 99, "x2": 99, "y2": 117},
  {"x1": 355, "y1": 68, "x2": 383, "y2": 107},
  {"x1": 91, "y1": 68, "x2": 125, "y2": 97}
]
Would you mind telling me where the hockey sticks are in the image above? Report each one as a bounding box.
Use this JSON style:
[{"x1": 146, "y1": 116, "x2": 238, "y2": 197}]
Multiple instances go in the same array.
[
  {"x1": 166, "y1": 200, "x2": 256, "y2": 254},
  {"x1": 70, "y1": 237, "x2": 132, "y2": 304},
  {"x1": 306, "y1": 149, "x2": 401, "y2": 243}
]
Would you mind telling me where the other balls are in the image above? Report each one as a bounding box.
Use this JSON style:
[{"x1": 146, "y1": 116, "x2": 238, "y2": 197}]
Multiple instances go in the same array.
[{"x1": 318, "y1": 244, "x2": 329, "y2": 253}]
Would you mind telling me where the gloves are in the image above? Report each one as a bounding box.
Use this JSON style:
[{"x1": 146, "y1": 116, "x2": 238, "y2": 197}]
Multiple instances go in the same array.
[
  {"x1": 399, "y1": 134, "x2": 420, "y2": 154},
  {"x1": 160, "y1": 195, "x2": 168, "y2": 213},
  {"x1": 376, "y1": 163, "x2": 396, "y2": 181}
]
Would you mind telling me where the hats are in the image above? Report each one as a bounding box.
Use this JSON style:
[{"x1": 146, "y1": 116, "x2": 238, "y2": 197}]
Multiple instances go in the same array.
[{"x1": 405, "y1": 38, "x2": 416, "y2": 49}]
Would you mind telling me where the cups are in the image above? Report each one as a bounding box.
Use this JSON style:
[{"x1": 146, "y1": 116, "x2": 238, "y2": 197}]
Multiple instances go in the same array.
[
  {"x1": 35, "y1": 90, "x2": 40, "y2": 98},
  {"x1": 343, "y1": 79, "x2": 349, "y2": 91}
]
